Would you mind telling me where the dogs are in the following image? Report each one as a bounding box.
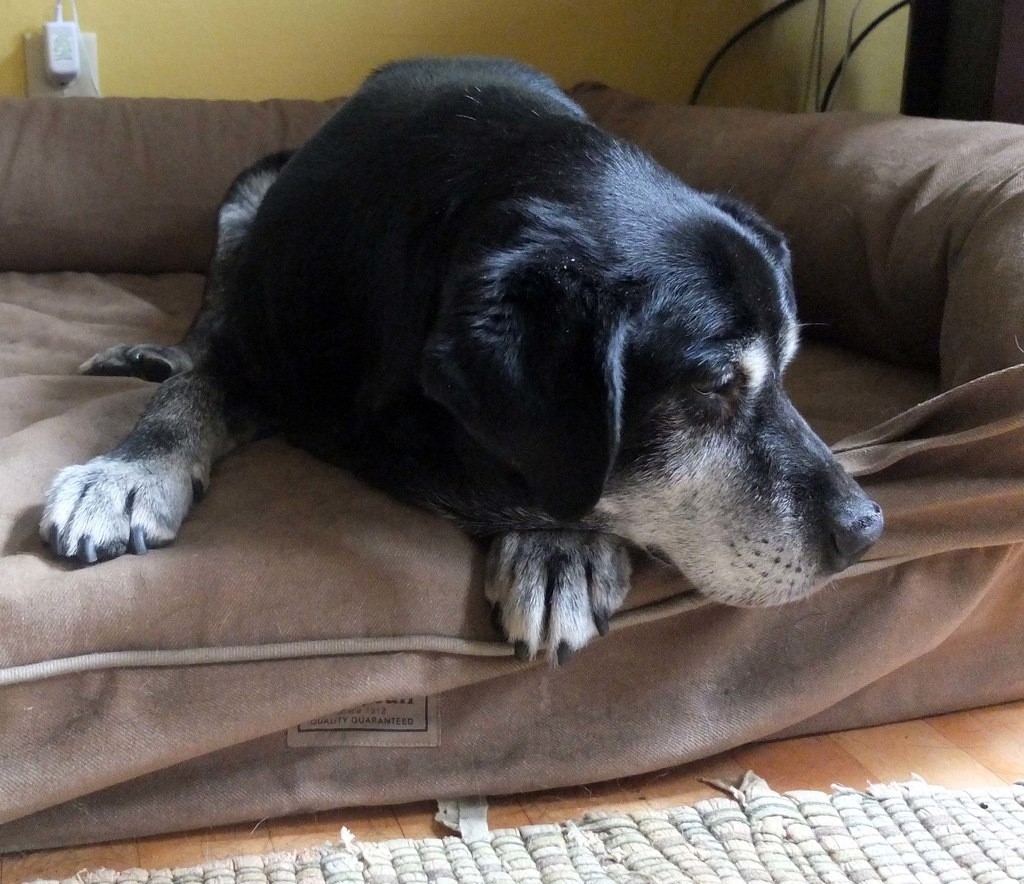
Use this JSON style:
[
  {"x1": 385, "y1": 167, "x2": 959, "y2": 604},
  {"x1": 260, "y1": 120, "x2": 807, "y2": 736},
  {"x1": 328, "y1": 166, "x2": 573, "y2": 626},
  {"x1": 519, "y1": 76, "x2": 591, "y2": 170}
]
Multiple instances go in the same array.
[{"x1": 32, "y1": 53, "x2": 886, "y2": 669}]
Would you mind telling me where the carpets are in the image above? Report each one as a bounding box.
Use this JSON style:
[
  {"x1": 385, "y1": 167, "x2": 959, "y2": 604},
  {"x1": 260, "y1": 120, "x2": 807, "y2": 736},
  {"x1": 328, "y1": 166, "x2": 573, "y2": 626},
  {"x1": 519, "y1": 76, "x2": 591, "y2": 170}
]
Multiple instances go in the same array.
[{"x1": 17, "y1": 769, "x2": 1024, "y2": 884}]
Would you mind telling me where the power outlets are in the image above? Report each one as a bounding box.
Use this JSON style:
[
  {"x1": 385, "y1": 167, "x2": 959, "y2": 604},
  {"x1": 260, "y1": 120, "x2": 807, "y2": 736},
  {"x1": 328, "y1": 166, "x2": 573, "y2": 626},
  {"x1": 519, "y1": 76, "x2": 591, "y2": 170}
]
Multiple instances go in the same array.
[{"x1": 24, "y1": 33, "x2": 100, "y2": 99}]
